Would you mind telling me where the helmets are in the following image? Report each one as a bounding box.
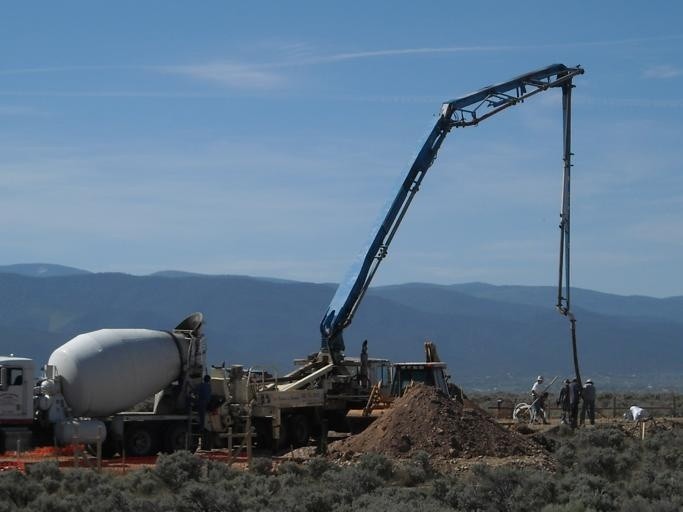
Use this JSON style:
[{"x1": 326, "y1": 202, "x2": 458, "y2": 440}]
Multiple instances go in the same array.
[
  {"x1": 586, "y1": 379, "x2": 594, "y2": 383},
  {"x1": 537, "y1": 376, "x2": 544, "y2": 381}
]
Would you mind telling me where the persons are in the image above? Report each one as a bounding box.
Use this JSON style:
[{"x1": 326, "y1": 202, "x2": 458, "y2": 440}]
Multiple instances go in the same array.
[
  {"x1": 568, "y1": 379, "x2": 580, "y2": 424},
  {"x1": 189, "y1": 375, "x2": 211, "y2": 430},
  {"x1": 531, "y1": 375, "x2": 550, "y2": 425},
  {"x1": 580, "y1": 381, "x2": 595, "y2": 424},
  {"x1": 557, "y1": 379, "x2": 570, "y2": 423}
]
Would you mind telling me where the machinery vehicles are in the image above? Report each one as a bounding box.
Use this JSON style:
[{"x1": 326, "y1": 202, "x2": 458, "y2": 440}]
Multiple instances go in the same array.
[{"x1": 200, "y1": 60, "x2": 590, "y2": 457}]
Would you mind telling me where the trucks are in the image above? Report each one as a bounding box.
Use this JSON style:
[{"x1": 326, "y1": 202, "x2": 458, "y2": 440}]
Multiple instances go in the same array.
[{"x1": 1, "y1": 313, "x2": 209, "y2": 457}]
